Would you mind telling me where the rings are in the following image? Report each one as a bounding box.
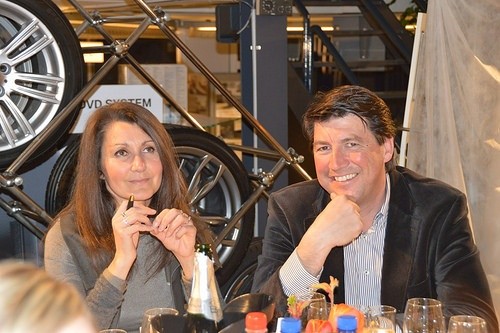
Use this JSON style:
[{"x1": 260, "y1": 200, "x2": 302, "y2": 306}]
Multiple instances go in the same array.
[
  {"x1": 122, "y1": 218, "x2": 131, "y2": 226},
  {"x1": 121, "y1": 210, "x2": 127, "y2": 217},
  {"x1": 182, "y1": 213, "x2": 192, "y2": 223},
  {"x1": 179, "y1": 209, "x2": 184, "y2": 214}
]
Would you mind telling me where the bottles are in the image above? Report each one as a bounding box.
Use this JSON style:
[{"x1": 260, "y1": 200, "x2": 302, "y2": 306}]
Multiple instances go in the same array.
[
  {"x1": 185, "y1": 243, "x2": 224, "y2": 333},
  {"x1": 245, "y1": 312, "x2": 269, "y2": 333},
  {"x1": 276, "y1": 317, "x2": 302, "y2": 333},
  {"x1": 337, "y1": 315, "x2": 357, "y2": 333}
]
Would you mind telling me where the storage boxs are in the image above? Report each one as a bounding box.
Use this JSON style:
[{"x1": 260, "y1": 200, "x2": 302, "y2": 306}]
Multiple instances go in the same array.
[{"x1": 68, "y1": 63, "x2": 188, "y2": 135}]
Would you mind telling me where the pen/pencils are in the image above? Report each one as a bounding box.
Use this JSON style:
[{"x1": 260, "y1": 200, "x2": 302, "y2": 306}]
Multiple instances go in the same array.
[{"x1": 125, "y1": 194, "x2": 134, "y2": 211}]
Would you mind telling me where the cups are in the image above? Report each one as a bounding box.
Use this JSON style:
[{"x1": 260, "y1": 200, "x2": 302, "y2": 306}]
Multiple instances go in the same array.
[
  {"x1": 296, "y1": 293, "x2": 326, "y2": 333},
  {"x1": 447, "y1": 315, "x2": 487, "y2": 333},
  {"x1": 309, "y1": 302, "x2": 335, "y2": 322},
  {"x1": 403, "y1": 298, "x2": 444, "y2": 333},
  {"x1": 365, "y1": 305, "x2": 396, "y2": 333},
  {"x1": 141, "y1": 307, "x2": 179, "y2": 333},
  {"x1": 99, "y1": 329, "x2": 128, "y2": 333}
]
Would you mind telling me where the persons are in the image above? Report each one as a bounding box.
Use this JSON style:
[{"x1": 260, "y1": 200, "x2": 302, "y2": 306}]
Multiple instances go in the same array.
[
  {"x1": 250, "y1": 85, "x2": 500, "y2": 333},
  {"x1": 43, "y1": 102, "x2": 224, "y2": 333},
  {"x1": 1, "y1": 258, "x2": 97, "y2": 333}
]
